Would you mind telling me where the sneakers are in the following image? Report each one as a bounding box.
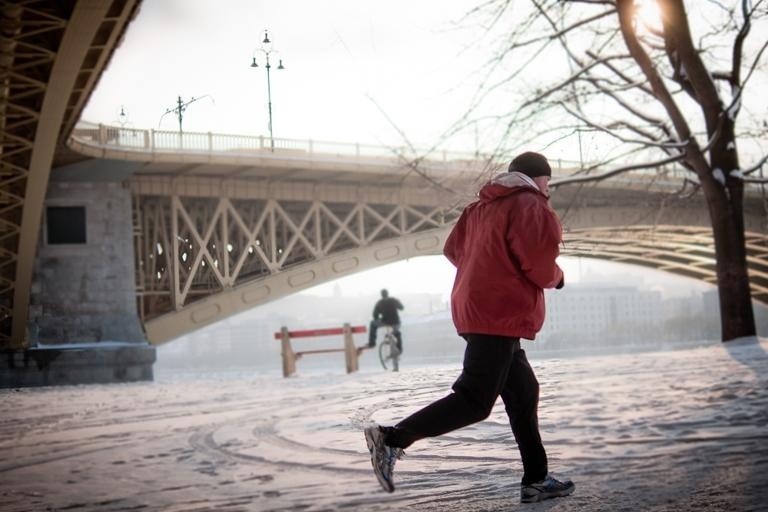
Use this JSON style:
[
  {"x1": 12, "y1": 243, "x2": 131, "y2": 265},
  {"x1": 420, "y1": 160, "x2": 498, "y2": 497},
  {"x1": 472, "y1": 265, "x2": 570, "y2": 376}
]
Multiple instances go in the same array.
[
  {"x1": 364, "y1": 425, "x2": 405, "y2": 493},
  {"x1": 520, "y1": 476, "x2": 576, "y2": 503}
]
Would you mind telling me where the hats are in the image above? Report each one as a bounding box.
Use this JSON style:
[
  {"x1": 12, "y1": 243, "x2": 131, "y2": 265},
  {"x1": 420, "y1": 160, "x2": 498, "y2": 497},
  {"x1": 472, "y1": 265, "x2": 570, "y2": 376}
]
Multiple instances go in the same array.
[{"x1": 509, "y1": 151, "x2": 552, "y2": 178}]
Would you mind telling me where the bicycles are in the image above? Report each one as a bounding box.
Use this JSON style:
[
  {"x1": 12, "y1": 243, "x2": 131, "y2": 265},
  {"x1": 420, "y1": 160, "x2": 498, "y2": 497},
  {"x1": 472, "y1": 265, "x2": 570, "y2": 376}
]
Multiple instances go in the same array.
[{"x1": 377, "y1": 316, "x2": 402, "y2": 371}]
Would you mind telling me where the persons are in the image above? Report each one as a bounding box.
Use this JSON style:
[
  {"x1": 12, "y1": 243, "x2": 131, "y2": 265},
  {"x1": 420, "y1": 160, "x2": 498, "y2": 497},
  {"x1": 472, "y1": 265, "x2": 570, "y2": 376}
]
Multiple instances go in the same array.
[
  {"x1": 364, "y1": 152, "x2": 575, "y2": 504},
  {"x1": 364, "y1": 288, "x2": 405, "y2": 353}
]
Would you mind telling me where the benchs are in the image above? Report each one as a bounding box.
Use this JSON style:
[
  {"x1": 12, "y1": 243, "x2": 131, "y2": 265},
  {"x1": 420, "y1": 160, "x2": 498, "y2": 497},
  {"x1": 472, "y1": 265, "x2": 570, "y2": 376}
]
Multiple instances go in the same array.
[{"x1": 274, "y1": 325, "x2": 370, "y2": 377}]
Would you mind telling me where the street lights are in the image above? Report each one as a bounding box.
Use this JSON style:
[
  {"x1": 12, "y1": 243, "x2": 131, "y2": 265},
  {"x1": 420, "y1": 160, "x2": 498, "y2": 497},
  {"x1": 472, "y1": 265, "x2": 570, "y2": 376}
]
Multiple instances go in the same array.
[
  {"x1": 566, "y1": 86, "x2": 588, "y2": 162},
  {"x1": 109, "y1": 104, "x2": 136, "y2": 145},
  {"x1": 251, "y1": 30, "x2": 284, "y2": 152}
]
